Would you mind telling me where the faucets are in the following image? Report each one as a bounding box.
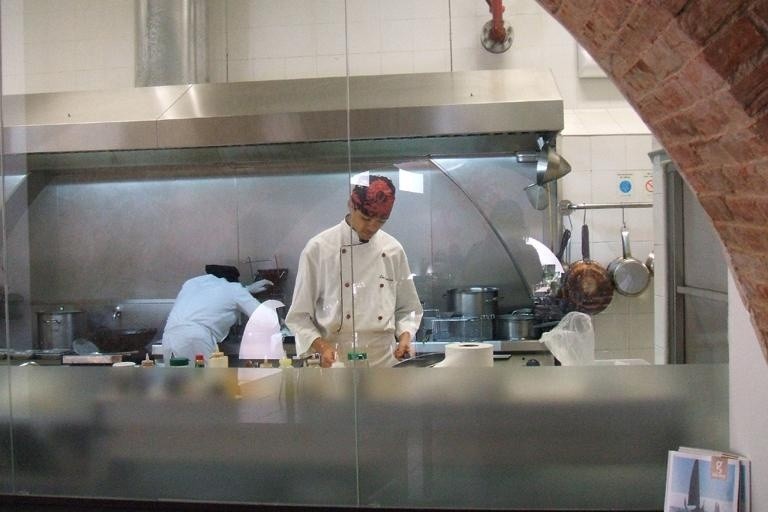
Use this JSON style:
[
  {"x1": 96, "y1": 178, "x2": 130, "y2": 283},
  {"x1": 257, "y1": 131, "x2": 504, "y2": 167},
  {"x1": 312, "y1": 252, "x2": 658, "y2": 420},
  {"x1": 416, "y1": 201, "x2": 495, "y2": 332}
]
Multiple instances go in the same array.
[{"x1": 111, "y1": 305, "x2": 121, "y2": 321}]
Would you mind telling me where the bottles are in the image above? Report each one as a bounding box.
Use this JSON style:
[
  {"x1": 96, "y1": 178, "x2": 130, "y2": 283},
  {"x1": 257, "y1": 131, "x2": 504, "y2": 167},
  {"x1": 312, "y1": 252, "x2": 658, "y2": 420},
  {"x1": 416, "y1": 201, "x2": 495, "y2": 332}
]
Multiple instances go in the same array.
[
  {"x1": 141, "y1": 352, "x2": 154, "y2": 368},
  {"x1": 194, "y1": 354, "x2": 205, "y2": 368}
]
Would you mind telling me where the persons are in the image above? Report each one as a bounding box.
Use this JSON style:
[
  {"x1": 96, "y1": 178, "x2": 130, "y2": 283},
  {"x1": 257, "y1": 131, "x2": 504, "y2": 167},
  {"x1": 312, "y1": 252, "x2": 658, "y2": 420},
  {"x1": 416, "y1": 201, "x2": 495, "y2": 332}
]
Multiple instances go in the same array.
[
  {"x1": 285, "y1": 175, "x2": 425, "y2": 370},
  {"x1": 162, "y1": 265, "x2": 284, "y2": 366}
]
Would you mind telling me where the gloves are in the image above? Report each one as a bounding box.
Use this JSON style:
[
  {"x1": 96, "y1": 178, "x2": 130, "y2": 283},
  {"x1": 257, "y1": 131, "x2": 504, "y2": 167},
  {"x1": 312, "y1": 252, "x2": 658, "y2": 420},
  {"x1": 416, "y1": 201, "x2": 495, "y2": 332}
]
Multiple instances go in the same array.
[{"x1": 246, "y1": 280, "x2": 274, "y2": 294}]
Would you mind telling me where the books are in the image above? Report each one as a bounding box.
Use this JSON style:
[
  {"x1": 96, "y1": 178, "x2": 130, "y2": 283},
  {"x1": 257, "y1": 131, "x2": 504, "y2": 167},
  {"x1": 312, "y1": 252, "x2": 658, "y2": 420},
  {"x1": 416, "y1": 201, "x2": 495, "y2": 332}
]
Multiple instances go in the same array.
[
  {"x1": 662, "y1": 449, "x2": 743, "y2": 511},
  {"x1": 677, "y1": 446, "x2": 752, "y2": 511}
]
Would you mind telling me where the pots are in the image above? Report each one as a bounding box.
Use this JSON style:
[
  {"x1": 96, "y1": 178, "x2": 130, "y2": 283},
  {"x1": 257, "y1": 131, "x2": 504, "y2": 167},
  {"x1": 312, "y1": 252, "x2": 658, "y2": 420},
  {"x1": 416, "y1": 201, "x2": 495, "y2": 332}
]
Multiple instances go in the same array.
[
  {"x1": 494, "y1": 310, "x2": 538, "y2": 340},
  {"x1": 607, "y1": 225, "x2": 651, "y2": 298},
  {"x1": 560, "y1": 224, "x2": 614, "y2": 316},
  {"x1": 35, "y1": 309, "x2": 83, "y2": 349},
  {"x1": 442, "y1": 286, "x2": 504, "y2": 314}
]
